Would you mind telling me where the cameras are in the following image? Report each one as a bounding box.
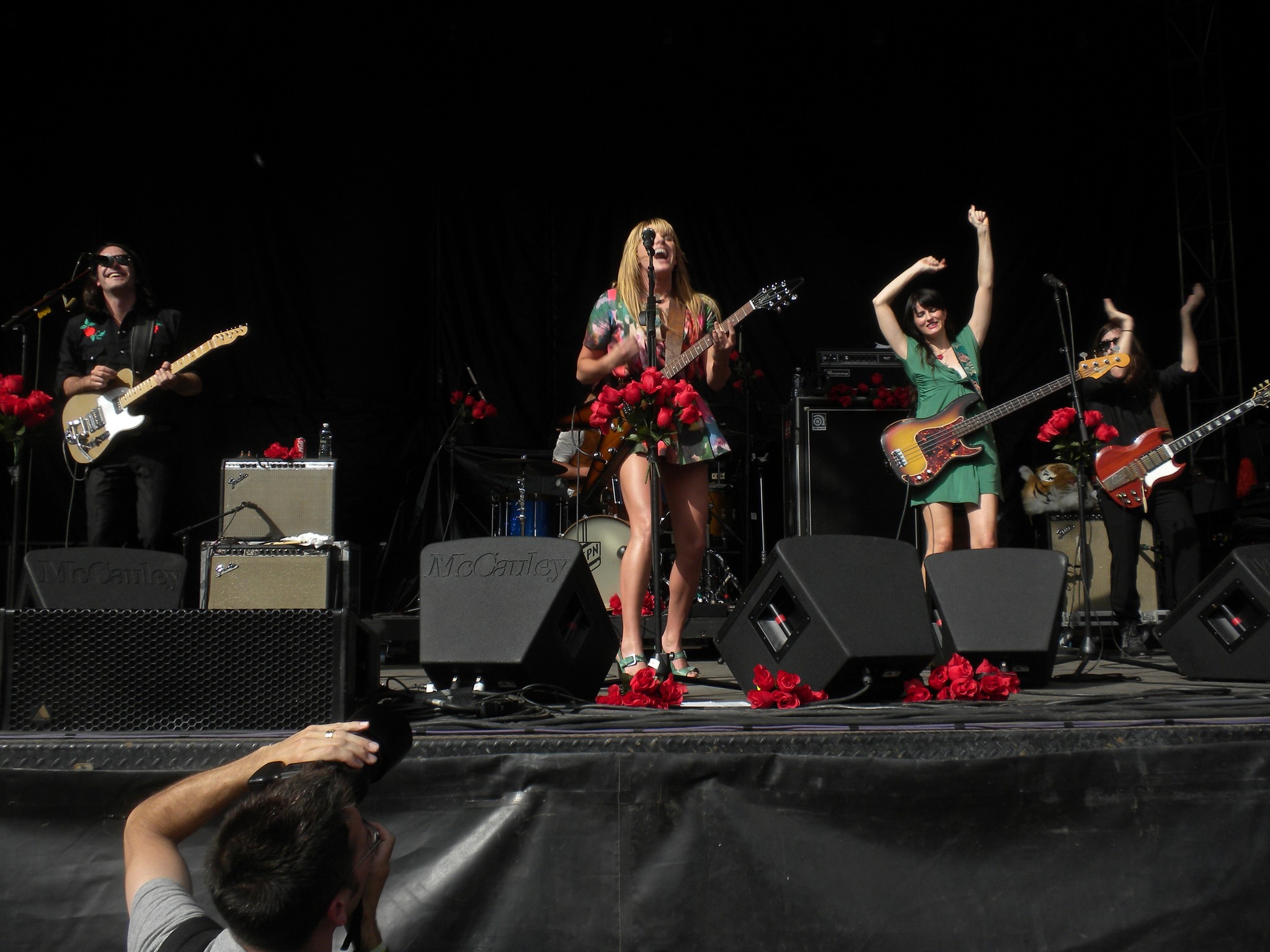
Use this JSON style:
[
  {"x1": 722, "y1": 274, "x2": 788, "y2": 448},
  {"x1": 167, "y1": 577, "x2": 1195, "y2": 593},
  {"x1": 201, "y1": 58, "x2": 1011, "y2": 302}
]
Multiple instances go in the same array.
[{"x1": 246, "y1": 693, "x2": 413, "y2": 801}]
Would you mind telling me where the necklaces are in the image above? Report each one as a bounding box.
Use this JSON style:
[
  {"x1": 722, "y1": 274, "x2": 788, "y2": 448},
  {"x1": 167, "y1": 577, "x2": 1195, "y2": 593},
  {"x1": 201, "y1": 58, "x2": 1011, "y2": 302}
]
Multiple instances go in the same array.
[{"x1": 935, "y1": 343, "x2": 951, "y2": 367}]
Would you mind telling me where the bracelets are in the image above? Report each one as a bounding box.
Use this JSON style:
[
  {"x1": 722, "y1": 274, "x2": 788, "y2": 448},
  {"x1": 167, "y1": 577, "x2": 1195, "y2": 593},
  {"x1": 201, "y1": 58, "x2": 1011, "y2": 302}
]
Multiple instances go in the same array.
[{"x1": 1120, "y1": 329, "x2": 1134, "y2": 333}]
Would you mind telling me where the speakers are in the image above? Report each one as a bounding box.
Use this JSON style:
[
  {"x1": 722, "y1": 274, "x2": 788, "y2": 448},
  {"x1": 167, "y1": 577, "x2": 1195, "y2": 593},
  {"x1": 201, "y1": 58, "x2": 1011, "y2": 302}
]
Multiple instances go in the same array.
[
  {"x1": 1154, "y1": 544, "x2": 1270, "y2": 681},
  {"x1": 0, "y1": 609, "x2": 383, "y2": 744},
  {"x1": 924, "y1": 546, "x2": 1067, "y2": 685},
  {"x1": 18, "y1": 548, "x2": 186, "y2": 610},
  {"x1": 199, "y1": 542, "x2": 359, "y2": 610},
  {"x1": 220, "y1": 457, "x2": 338, "y2": 542},
  {"x1": 713, "y1": 535, "x2": 936, "y2": 705},
  {"x1": 418, "y1": 537, "x2": 620, "y2": 707},
  {"x1": 1047, "y1": 511, "x2": 1168, "y2": 628}
]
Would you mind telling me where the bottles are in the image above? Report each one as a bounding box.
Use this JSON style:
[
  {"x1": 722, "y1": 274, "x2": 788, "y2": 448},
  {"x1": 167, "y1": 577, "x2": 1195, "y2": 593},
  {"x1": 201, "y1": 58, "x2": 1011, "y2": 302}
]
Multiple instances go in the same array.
[{"x1": 318, "y1": 423, "x2": 332, "y2": 460}]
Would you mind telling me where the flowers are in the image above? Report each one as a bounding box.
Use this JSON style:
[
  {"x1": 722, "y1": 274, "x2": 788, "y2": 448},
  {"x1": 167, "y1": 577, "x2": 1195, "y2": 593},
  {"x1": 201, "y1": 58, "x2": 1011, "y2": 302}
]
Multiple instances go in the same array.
[
  {"x1": 589, "y1": 366, "x2": 702, "y2": 484},
  {"x1": 608, "y1": 589, "x2": 667, "y2": 617},
  {"x1": 730, "y1": 350, "x2": 764, "y2": 389},
  {"x1": 824, "y1": 373, "x2": 918, "y2": 409},
  {"x1": 1037, "y1": 409, "x2": 1120, "y2": 481},
  {"x1": 746, "y1": 664, "x2": 828, "y2": 709},
  {"x1": 0, "y1": 373, "x2": 55, "y2": 485},
  {"x1": 263, "y1": 444, "x2": 302, "y2": 459},
  {"x1": 446, "y1": 391, "x2": 496, "y2": 435},
  {"x1": 900, "y1": 651, "x2": 1021, "y2": 703},
  {"x1": 596, "y1": 668, "x2": 688, "y2": 713}
]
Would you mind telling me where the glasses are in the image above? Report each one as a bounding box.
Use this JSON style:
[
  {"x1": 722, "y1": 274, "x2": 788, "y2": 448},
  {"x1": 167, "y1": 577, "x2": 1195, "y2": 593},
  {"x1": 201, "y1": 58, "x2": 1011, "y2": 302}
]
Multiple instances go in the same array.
[
  {"x1": 114, "y1": 255, "x2": 129, "y2": 266},
  {"x1": 1098, "y1": 336, "x2": 1121, "y2": 351},
  {"x1": 321, "y1": 817, "x2": 382, "y2": 916}
]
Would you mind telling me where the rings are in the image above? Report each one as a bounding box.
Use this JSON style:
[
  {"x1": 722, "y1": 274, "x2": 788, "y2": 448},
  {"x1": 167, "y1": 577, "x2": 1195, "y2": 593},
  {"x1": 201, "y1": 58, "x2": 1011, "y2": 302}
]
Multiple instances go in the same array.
[{"x1": 325, "y1": 730, "x2": 336, "y2": 738}]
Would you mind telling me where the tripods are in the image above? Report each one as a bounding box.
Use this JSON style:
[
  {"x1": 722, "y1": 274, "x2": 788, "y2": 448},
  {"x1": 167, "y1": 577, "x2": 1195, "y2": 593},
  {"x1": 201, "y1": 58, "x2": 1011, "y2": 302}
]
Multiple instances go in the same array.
[
  {"x1": 1052, "y1": 292, "x2": 1183, "y2": 680},
  {"x1": 600, "y1": 253, "x2": 741, "y2": 698}
]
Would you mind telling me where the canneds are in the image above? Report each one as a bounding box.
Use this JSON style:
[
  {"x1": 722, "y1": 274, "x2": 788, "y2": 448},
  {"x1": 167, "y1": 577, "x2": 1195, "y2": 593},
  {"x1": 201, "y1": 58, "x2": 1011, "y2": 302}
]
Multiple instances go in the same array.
[{"x1": 295, "y1": 437, "x2": 306, "y2": 458}]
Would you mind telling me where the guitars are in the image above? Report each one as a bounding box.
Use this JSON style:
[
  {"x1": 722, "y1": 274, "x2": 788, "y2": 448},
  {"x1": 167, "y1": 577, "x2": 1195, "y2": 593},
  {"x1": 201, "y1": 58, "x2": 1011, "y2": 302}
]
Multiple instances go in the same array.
[
  {"x1": 880, "y1": 343, "x2": 1131, "y2": 486},
  {"x1": 552, "y1": 279, "x2": 800, "y2": 504},
  {"x1": 1094, "y1": 380, "x2": 1270, "y2": 509},
  {"x1": 60, "y1": 322, "x2": 250, "y2": 465}
]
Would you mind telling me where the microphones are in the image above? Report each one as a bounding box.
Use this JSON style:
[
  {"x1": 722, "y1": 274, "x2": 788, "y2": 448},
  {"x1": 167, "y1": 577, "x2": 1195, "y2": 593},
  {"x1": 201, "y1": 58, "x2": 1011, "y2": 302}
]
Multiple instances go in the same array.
[
  {"x1": 641, "y1": 227, "x2": 656, "y2": 251},
  {"x1": 1043, "y1": 273, "x2": 1066, "y2": 289},
  {"x1": 466, "y1": 364, "x2": 488, "y2": 407},
  {"x1": 81, "y1": 252, "x2": 115, "y2": 268}
]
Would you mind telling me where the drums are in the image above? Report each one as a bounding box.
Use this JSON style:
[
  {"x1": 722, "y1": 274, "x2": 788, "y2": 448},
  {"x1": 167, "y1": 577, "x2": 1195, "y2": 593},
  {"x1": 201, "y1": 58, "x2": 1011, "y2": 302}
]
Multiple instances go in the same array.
[
  {"x1": 558, "y1": 513, "x2": 649, "y2": 613},
  {"x1": 492, "y1": 491, "x2": 568, "y2": 539}
]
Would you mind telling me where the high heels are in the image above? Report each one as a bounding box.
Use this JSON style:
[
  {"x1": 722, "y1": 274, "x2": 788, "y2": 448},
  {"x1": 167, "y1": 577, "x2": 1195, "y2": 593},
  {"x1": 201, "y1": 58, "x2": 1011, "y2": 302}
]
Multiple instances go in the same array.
[
  {"x1": 660, "y1": 645, "x2": 699, "y2": 680},
  {"x1": 615, "y1": 642, "x2": 648, "y2": 687}
]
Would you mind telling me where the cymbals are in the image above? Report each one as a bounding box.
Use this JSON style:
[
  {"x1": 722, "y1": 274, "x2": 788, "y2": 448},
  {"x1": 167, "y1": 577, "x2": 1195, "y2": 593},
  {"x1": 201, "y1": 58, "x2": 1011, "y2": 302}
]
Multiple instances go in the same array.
[{"x1": 478, "y1": 458, "x2": 568, "y2": 480}]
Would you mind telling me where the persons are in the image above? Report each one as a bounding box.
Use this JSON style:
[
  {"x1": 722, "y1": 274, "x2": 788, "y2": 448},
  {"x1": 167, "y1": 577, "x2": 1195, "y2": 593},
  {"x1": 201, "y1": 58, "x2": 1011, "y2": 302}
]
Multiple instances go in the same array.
[
  {"x1": 872, "y1": 205, "x2": 1005, "y2": 592},
  {"x1": 123, "y1": 722, "x2": 396, "y2": 952},
  {"x1": 56, "y1": 242, "x2": 205, "y2": 551},
  {"x1": 1084, "y1": 283, "x2": 1204, "y2": 659},
  {"x1": 552, "y1": 429, "x2": 601, "y2": 499},
  {"x1": 575, "y1": 220, "x2": 736, "y2": 690}
]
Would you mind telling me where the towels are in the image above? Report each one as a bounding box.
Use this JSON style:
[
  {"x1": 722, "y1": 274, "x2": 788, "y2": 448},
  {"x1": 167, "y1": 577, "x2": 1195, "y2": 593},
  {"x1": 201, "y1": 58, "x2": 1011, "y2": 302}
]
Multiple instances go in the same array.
[{"x1": 280, "y1": 531, "x2": 334, "y2": 548}]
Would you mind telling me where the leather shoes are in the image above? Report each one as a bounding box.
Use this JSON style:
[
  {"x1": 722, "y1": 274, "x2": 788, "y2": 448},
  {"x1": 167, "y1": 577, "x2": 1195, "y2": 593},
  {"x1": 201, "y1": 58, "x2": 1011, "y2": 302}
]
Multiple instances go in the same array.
[{"x1": 1121, "y1": 627, "x2": 1154, "y2": 658}]
